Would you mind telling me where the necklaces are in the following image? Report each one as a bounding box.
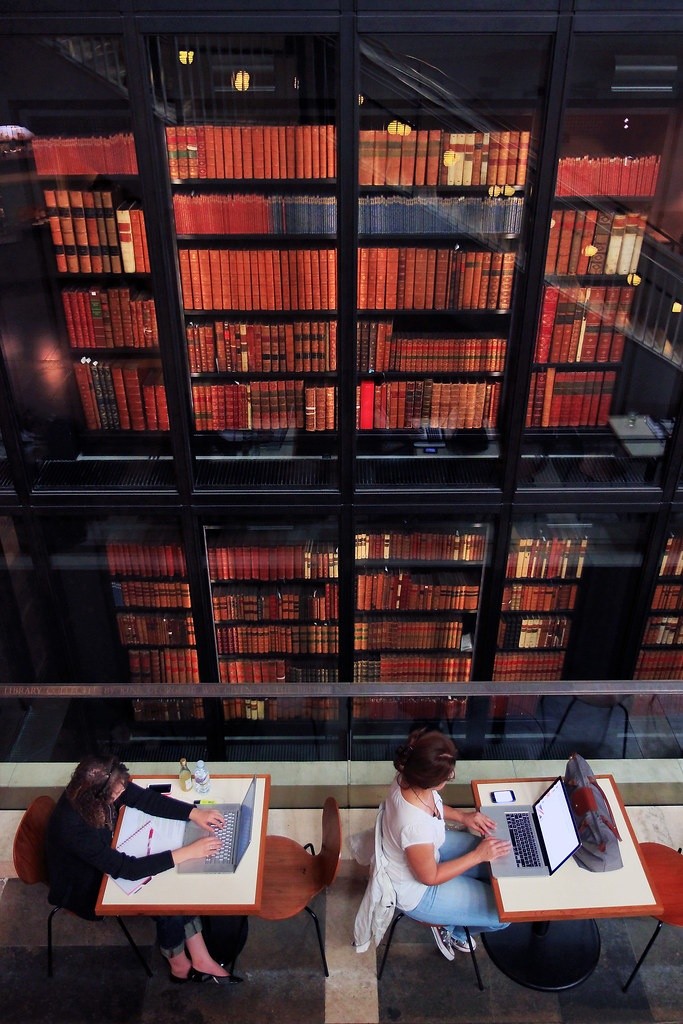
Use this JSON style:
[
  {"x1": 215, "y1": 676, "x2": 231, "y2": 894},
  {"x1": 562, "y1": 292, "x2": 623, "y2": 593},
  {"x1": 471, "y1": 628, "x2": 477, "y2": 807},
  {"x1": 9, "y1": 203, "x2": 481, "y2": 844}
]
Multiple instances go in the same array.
[{"x1": 410, "y1": 787, "x2": 440, "y2": 818}]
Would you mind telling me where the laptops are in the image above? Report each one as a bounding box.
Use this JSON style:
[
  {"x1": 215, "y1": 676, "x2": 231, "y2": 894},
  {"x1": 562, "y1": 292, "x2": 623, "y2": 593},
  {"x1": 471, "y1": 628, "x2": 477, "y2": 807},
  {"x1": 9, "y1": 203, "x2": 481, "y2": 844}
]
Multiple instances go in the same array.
[
  {"x1": 480, "y1": 775, "x2": 583, "y2": 878},
  {"x1": 175, "y1": 774, "x2": 257, "y2": 873}
]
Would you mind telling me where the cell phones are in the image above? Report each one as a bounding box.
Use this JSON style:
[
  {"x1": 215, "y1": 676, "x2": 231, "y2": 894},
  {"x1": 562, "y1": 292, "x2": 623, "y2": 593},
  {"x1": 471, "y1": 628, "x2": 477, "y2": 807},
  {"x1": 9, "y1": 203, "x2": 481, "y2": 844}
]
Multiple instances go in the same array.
[
  {"x1": 490, "y1": 790, "x2": 516, "y2": 803},
  {"x1": 147, "y1": 783, "x2": 173, "y2": 795}
]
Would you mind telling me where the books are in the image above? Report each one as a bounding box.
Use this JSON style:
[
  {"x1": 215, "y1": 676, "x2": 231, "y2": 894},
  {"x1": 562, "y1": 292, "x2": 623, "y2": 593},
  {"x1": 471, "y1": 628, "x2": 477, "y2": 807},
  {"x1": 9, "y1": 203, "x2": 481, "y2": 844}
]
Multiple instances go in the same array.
[
  {"x1": 26, "y1": 125, "x2": 668, "y2": 489},
  {"x1": 101, "y1": 521, "x2": 683, "y2": 762}
]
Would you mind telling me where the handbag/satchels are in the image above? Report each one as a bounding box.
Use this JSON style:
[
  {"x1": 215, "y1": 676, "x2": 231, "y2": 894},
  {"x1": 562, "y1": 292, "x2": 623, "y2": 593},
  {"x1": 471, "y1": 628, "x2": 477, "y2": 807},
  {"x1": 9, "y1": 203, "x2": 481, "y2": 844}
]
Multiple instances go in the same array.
[{"x1": 564, "y1": 753, "x2": 624, "y2": 873}]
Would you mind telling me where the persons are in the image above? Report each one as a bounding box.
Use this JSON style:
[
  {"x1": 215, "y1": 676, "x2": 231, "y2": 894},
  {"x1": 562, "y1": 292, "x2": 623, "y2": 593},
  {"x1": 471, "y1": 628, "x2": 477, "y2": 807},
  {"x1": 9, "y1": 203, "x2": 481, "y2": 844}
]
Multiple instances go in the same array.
[
  {"x1": 38, "y1": 756, "x2": 246, "y2": 987},
  {"x1": 380, "y1": 729, "x2": 512, "y2": 962}
]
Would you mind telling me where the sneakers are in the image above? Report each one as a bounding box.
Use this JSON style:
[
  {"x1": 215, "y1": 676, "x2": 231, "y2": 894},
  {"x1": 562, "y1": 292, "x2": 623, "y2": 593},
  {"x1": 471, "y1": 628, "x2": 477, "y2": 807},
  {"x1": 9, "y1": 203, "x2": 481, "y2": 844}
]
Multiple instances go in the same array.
[
  {"x1": 451, "y1": 932, "x2": 476, "y2": 952},
  {"x1": 431, "y1": 927, "x2": 455, "y2": 961}
]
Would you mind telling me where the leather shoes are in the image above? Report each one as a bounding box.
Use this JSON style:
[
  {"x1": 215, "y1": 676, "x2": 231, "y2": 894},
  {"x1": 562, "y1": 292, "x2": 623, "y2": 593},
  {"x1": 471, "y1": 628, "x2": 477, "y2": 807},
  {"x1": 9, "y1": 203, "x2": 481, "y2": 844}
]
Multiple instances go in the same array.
[{"x1": 169, "y1": 966, "x2": 244, "y2": 986}]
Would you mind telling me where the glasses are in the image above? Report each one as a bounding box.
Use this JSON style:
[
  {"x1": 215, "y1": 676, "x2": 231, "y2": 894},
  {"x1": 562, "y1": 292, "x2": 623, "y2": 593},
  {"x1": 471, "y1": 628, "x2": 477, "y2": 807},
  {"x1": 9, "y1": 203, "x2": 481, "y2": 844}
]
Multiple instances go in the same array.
[{"x1": 444, "y1": 771, "x2": 455, "y2": 782}]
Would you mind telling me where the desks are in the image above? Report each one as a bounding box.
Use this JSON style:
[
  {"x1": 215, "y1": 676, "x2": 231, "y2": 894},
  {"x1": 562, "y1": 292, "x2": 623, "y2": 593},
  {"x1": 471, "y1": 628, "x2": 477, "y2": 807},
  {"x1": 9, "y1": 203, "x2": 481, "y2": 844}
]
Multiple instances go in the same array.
[
  {"x1": 97, "y1": 769, "x2": 272, "y2": 981},
  {"x1": 470, "y1": 776, "x2": 663, "y2": 991}
]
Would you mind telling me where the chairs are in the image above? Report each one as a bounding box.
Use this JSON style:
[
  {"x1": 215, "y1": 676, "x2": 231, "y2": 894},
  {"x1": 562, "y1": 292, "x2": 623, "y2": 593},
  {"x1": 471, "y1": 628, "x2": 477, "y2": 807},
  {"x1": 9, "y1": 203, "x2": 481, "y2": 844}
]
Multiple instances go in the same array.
[
  {"x1": 13, "y1": 792, "x2": 156, "y2": 978},
  {"x1": 228, "y1": 799, "x2": 341, "y2": 984},
  {"x1": 349, "y1": 802, "x2": 484, "y2": 990},
  {"x1": 616, "y1": 839, "x2": 683, "y2": 992}
]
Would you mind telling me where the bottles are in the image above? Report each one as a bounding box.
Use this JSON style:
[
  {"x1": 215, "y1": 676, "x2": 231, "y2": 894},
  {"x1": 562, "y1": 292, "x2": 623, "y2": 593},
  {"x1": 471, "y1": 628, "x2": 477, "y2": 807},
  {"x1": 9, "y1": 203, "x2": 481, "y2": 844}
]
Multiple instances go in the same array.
[
  {"x1": 179, "y1": 758, "x2": 192, "y2": 791},
  {"x1": 629, "y1": 415, "x2": 635, "y2": 427},
  {"x1": 195, "y1": 760, "x2": 210, "y2": 793}
]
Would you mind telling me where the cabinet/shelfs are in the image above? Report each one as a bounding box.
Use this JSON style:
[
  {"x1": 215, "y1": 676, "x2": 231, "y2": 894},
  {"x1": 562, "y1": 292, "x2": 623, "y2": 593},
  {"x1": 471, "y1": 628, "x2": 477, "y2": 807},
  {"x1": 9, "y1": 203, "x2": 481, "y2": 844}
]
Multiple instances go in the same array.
[{"x1": 0, "y1": 98, "x2": 682, "y2": 723}]
